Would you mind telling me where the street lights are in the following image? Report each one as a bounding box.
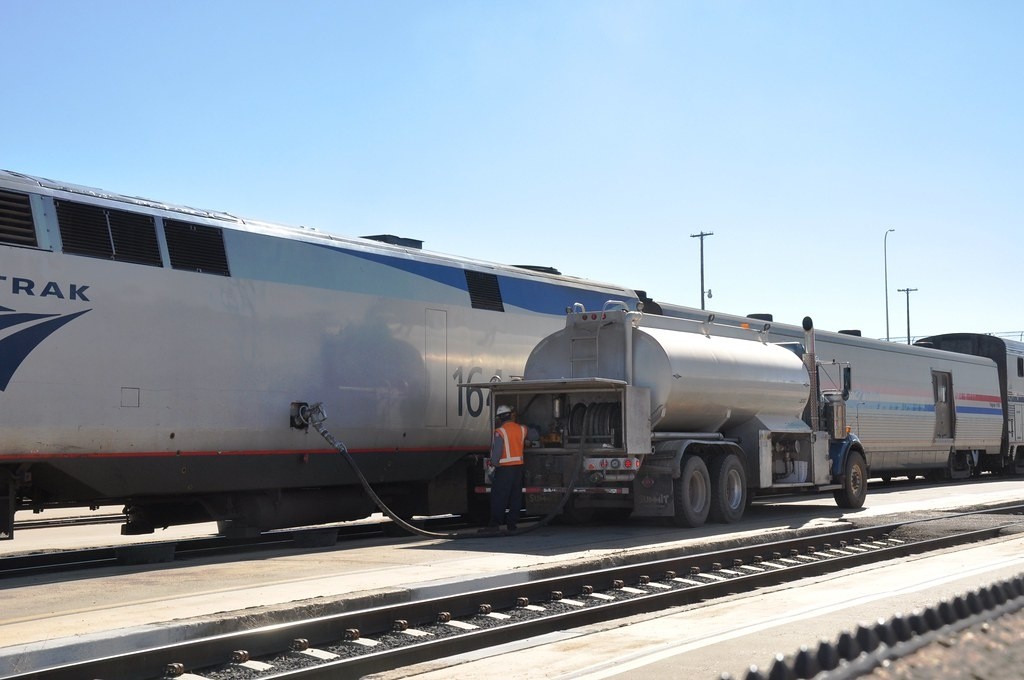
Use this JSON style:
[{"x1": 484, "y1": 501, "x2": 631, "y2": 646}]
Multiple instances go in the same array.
[{"x1": 884, "y1": 229, "x2": 896, "y2": 342}]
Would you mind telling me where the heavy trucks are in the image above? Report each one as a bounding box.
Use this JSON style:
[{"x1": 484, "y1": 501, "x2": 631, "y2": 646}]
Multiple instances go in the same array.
[{"x1": 457, "y1": 298, "x2": 870, "y2": 527}]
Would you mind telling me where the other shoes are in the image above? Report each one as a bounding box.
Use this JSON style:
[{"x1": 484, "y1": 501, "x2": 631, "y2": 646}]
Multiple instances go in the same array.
[
  {"x1": 477, "y1": 525, "x2": 499, "y2": 532},
  {"x1": 507, "y1": 523, "x2": 516, "y2": 530}
]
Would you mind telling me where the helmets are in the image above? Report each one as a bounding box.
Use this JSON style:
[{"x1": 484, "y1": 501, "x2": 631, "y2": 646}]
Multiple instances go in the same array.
[{"x1": 497, "y1": 405, "x2": 511, "y2": 415}]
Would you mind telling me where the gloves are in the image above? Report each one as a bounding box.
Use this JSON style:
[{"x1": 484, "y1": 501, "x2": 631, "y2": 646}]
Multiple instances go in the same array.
[{"x1": 487, "y1": 466, "x2": 496, "y2": 475}]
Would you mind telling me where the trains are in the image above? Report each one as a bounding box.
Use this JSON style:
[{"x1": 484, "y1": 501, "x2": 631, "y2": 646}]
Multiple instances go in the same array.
[{"x1": 0, "y1": 165, "x2": 1023, "y2": 535}]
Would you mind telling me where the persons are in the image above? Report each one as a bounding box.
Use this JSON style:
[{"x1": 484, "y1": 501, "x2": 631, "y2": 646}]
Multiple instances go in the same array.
[{"x1": 477, "y1": 406, "x2": 529, "y2": 532}]
